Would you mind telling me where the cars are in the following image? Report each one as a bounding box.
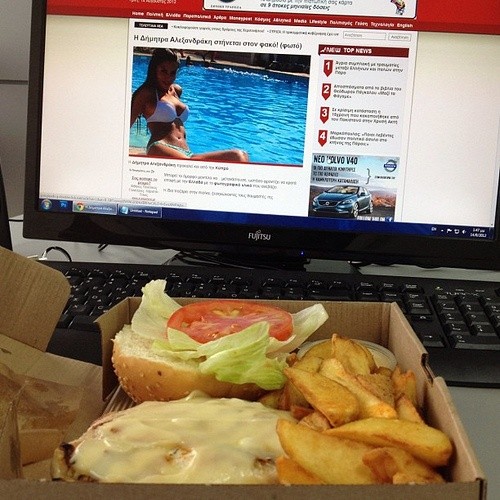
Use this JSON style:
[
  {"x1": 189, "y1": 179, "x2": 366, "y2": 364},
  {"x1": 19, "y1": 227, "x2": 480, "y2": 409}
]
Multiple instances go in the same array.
[{"x1": 313, "y1": 184, "x2": 374, "y2": 218}]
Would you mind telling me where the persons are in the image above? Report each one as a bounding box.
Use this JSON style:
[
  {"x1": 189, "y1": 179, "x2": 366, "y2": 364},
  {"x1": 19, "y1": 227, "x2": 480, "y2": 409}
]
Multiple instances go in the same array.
[{"x1": 131, "y1": 49, "x2": 248, "y2": 163}]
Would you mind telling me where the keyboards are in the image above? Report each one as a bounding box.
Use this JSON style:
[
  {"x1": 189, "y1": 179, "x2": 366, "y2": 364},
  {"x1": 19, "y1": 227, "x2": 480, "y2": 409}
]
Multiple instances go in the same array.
[{"x1": 36, "y1": 258, "x2": 500, "y2": 388}]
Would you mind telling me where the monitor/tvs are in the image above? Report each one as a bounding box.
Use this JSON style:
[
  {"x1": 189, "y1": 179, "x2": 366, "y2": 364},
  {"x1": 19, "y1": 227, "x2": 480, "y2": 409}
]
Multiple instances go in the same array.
[{"x1": 21, "y1": 1, "x2": 500, "y2": 271}]
